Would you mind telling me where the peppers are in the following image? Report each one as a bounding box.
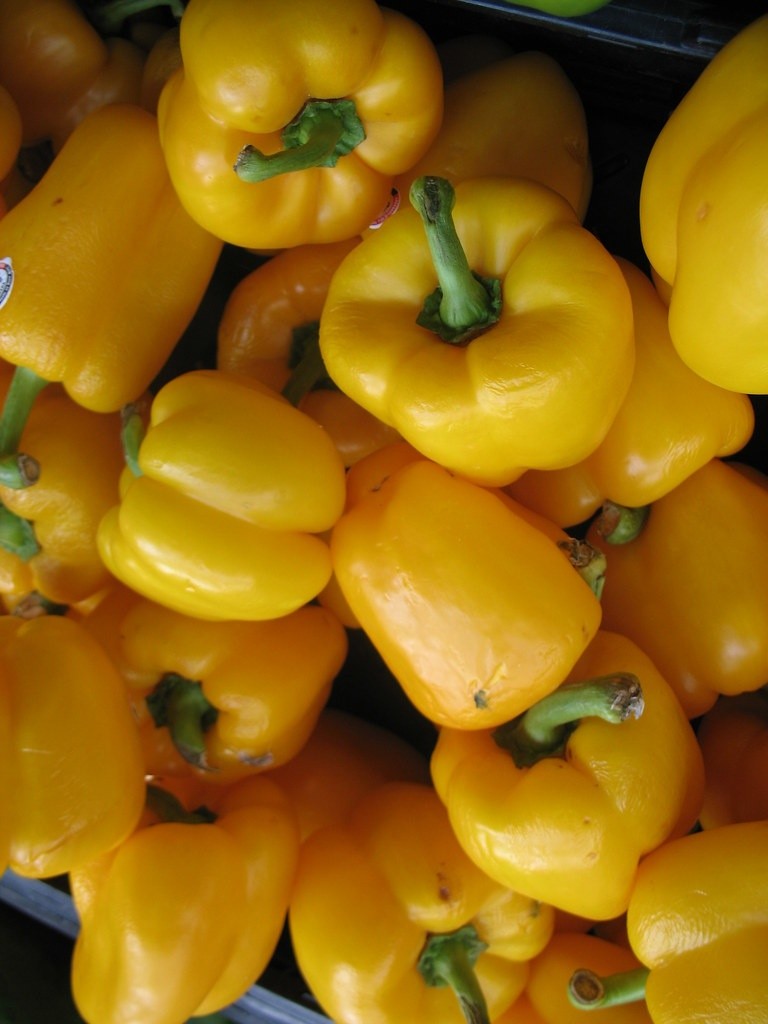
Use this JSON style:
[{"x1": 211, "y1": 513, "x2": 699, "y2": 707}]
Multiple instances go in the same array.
[{"x1": 0, "y1": 0, "x2": 768, "y2": 1024}]
